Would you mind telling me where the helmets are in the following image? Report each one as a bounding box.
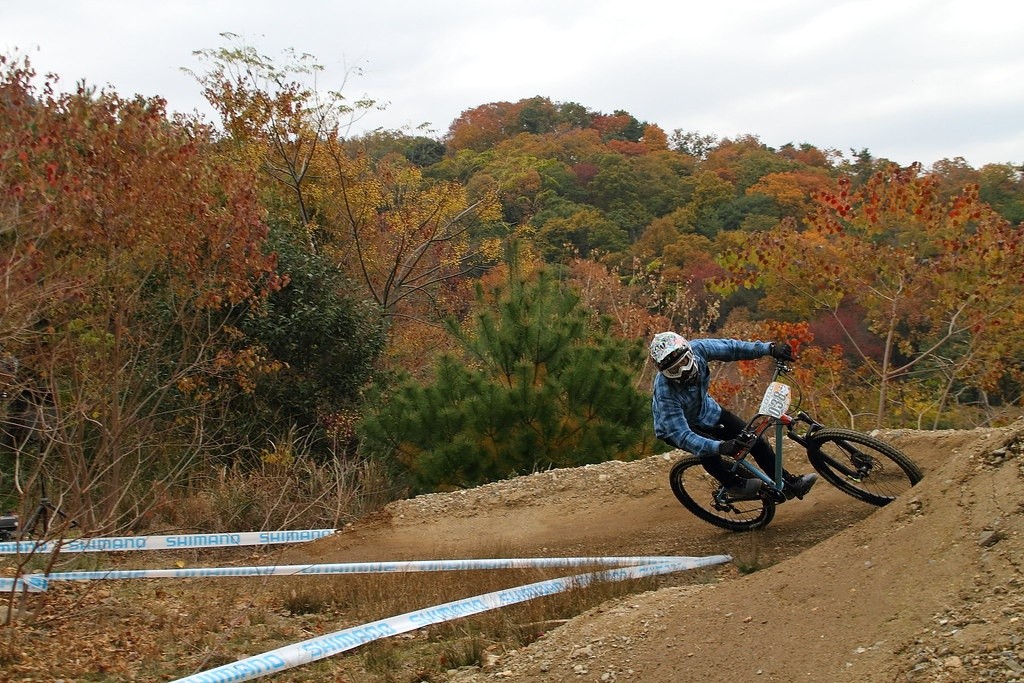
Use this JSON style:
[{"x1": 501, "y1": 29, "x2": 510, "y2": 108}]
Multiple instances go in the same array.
[{"x1": 649, "y1": 331, "x2": 700, "y2": 389}]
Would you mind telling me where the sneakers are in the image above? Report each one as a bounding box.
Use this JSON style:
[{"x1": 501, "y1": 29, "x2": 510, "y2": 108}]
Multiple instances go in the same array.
[
  {"x1": 782, "y1": 472, "x2": 818, "y2": 500},
  {"x1": 725, "y1": 478, "x2": 763, "y2": 496}
]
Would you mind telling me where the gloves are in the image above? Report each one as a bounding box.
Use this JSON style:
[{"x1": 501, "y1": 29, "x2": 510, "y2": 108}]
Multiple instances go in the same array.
[
  {"x1": 719, "y1": 438, "x2": 751, "y2": 455},
  {"x1": 770, "y1": 343, "x2": 795, "y2": 363}
]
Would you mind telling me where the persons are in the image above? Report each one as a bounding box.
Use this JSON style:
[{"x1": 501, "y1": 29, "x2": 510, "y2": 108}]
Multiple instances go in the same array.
[{"x1": 650, "y1": 331, "x2": 818, "y2": 502}]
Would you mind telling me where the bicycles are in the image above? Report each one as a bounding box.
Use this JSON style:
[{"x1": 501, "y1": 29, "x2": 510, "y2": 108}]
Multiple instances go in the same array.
[{"x1": 669, "y1": 357, "x2": 923, "y2": 533}]
